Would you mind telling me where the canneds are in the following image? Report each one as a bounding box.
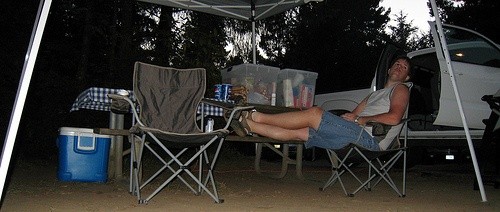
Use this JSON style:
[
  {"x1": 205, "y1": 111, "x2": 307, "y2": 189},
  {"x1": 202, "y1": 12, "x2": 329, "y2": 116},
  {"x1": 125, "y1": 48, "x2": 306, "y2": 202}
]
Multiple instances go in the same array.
[{"x1": 214, "y1": 84, "x2": 232, "y2": 102}]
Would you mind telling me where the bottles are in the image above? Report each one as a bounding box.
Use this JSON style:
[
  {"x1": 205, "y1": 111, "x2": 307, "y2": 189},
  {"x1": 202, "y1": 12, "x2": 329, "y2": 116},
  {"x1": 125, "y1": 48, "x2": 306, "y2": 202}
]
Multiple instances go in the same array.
[{"x1": 206, "y1": 117, "x2": 214, "y2": 133}]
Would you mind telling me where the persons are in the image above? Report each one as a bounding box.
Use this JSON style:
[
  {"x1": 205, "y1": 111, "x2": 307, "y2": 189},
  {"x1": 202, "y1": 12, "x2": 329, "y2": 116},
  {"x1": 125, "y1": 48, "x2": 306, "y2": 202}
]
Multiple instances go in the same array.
[{"x1": 224, "y1": 55, "x2": 411, "y2": 154}]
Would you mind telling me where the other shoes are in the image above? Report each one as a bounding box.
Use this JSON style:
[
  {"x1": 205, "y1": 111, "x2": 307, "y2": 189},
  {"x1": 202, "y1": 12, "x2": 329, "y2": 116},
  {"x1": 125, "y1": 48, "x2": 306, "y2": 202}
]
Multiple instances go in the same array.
[{"x1": 224, "y1": 106, "x2": 255, "y2": 137}]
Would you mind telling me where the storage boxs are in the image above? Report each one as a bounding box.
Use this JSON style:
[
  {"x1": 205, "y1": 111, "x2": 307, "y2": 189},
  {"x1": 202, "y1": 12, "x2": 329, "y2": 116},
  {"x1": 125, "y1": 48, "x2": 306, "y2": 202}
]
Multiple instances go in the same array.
[
  {"x1": 220, "y1": 64, "x2": 281, "y2": 106},
  {"x1": 56, "y1": 126, "x2": 111, "y2": 182},
  {"x1": 278, "y1": 68, "x2": 318, "y2": 108}
]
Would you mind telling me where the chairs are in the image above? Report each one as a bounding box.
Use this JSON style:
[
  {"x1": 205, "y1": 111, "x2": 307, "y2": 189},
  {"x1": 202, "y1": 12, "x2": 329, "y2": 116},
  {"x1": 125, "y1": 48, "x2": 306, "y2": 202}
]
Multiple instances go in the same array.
[
  {"x1": 318, "y1": 81, "x2": 413, "y2": 198},
  {"x1": 107, "y1": 62, "x2": 254, "y2": 211}
]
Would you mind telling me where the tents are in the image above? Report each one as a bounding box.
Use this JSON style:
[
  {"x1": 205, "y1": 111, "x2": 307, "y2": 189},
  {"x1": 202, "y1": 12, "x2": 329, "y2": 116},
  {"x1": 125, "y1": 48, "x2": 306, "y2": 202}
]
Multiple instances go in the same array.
[{"x1": 0, "y1": 0, "x2": 488, "y2": 202}]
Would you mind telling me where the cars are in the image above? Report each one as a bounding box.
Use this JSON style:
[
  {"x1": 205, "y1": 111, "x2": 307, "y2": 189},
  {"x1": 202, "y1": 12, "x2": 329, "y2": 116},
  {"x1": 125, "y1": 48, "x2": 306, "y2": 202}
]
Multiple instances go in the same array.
[{"x1": 269, "y1": 20, "x2": 500, "y2": 169}]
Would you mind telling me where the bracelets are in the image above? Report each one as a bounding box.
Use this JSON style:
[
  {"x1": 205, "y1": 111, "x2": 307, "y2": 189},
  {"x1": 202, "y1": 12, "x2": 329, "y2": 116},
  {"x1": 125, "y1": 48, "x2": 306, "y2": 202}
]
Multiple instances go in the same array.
[{"x1": 352, "y1": 114, "x2": 360, "y2": 124}]
